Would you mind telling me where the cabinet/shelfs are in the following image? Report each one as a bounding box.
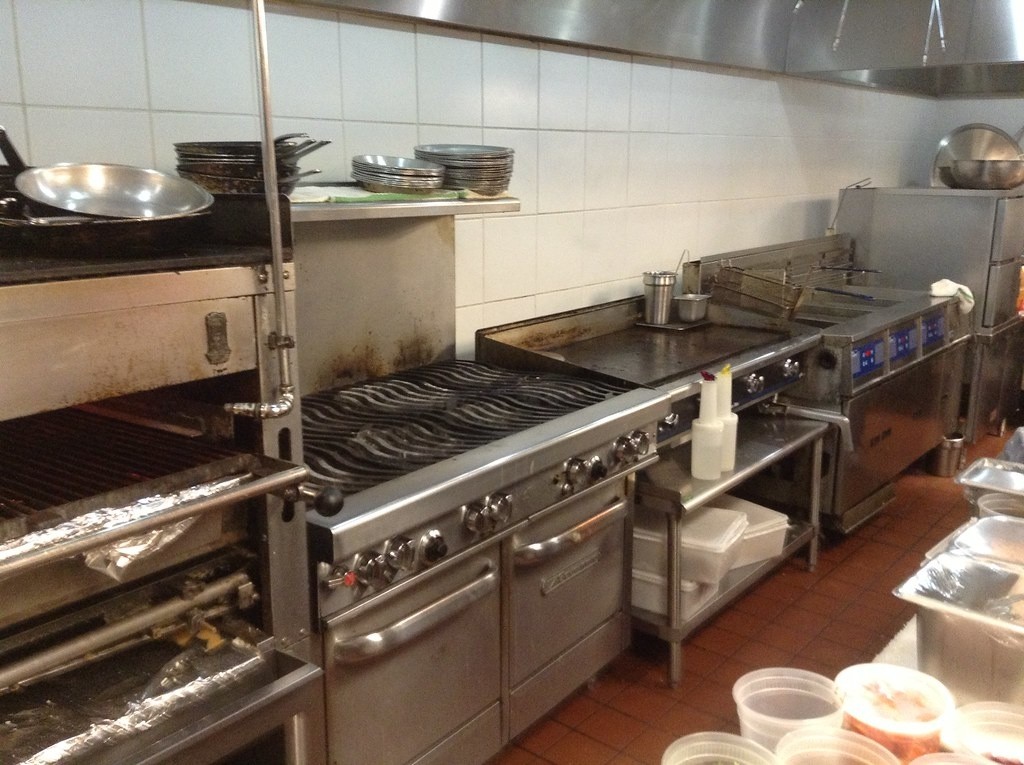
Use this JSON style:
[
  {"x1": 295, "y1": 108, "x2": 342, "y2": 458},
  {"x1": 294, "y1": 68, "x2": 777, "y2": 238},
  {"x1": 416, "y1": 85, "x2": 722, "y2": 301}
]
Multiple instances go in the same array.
[{"x1": 632, "y1": 415, "x2": 829, "y2": 685}]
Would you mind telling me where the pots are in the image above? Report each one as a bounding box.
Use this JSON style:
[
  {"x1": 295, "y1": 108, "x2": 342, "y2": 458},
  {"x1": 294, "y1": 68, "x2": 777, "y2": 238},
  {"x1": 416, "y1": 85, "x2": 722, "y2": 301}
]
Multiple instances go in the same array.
[{"x1": 174, "y1": 132, "x2": 332, "y2": 195}]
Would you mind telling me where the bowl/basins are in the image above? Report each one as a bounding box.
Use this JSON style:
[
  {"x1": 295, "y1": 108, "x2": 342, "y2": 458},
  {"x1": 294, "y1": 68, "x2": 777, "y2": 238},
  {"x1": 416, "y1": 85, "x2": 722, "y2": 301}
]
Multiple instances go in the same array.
[
  {"x1": 949, "y1": 159, "x2": 1024, "y2": 190},
  {"x1": 672, "y1": 294, "x2": 712, "y2": 322},
  {"x1": 938, "y1": 166, "x2": 957, "y2": 189}
]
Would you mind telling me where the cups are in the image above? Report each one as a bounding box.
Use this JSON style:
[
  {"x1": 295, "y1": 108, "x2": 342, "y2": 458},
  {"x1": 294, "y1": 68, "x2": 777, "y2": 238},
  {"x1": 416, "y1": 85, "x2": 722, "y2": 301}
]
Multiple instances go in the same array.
[
  {"x1": 643, "y1": 271, "x2": 678, "y2": 325},
  {"x1": 775, "y1": 727, "x2": 901, "y2": 765},
  {"x1": 732, "y1": 668, "x2": 845, "y2": 753},
  {"x1": 661, "y1": 732, "x2": 775, "y2": 765},
  {"x1": 909, "y1": 753, "x2": 1003, "y2": 765},
  {"x1": 836, "y1": 663, "x2": 955, "y2": 765},
  {"x1": 940, "y1": 703, "x2": 1024, "y2": 765},
  {"x1": 977, "y1": 494, "x2": 1024, "y2": 519}
]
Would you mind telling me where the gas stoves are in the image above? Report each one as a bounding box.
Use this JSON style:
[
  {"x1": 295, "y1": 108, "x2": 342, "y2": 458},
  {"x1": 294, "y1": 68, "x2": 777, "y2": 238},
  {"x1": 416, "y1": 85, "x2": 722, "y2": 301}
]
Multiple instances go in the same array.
[{"x1": 300, "y1": 359, "x2": 671, "y2": 617}]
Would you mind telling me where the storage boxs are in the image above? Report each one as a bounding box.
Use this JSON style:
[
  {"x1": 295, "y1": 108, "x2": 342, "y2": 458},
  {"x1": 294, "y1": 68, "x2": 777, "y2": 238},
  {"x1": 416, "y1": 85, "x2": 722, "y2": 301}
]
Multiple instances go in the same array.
[
  {"x1": 632, "y1": 502, "x2": 749, "y2": 585},
  {"x1": 703, "y1": 494, "x2": 791, "y2": 571},
  {"x1": 632, "y1": 569, "x2": 719, "y2": 620}
]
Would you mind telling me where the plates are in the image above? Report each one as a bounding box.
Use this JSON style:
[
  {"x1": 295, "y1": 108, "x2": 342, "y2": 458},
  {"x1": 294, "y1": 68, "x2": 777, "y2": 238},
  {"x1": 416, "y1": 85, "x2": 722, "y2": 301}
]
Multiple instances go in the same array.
[
  {"x1": 636, "y1": 319, "x2": 710, "y2": 331},
  {"x1": 15, "y1": 162, "x2": 214, "y2": 218},
  {"x1": 352, "y1": 143, "x2": 515, "y2": 197}
]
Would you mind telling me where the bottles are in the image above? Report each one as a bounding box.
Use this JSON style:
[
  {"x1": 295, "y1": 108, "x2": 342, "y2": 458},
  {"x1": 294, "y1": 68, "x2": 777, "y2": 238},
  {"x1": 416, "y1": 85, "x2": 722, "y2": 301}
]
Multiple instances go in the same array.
[
  {"x1": 717, "y1": 371, "x2": 739, "y2": 472},
  {"x1": 691, "y1": 379, "x2": 724, "y2": 479}
]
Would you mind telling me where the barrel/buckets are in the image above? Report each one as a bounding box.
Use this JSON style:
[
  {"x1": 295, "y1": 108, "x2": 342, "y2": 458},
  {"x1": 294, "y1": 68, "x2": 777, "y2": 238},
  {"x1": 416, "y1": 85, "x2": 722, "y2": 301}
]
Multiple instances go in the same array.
[{"x1": 925, "y1": 433, "x2": 966, "y2": 477}]
